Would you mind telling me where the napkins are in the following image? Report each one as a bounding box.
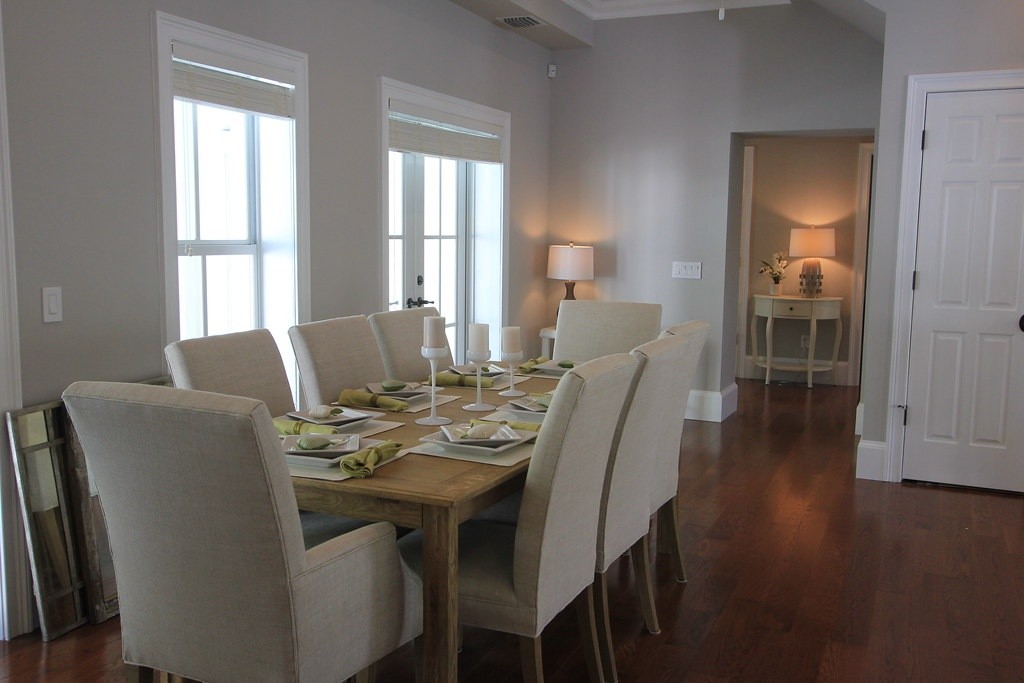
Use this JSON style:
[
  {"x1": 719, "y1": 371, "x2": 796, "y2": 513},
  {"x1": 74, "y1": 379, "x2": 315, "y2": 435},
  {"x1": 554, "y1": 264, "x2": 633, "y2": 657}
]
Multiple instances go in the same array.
[
  {"x1": 426, "y1": 371, "x2": 494, "y2": 388},
  {"x1": 338, "y1": 388, "x2": 408, "y2": 412},
  {"x1": 518, "y1": 355, "x2": 549, "y2": 374},
  {"x1": 469, "y1": 417, "x2": 542, "y2": 433},
  {"x1": 272, "y1": 417, "x2": 339, "y2": 435},
  {"x1": 340, "y1": 439, "x2": 404, "y2": 479}
]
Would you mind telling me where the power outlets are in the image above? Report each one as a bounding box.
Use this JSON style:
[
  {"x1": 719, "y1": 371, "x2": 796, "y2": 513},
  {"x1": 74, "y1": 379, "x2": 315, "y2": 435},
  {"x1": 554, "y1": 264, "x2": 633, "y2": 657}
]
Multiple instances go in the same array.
[{"x1": 800, "y1": 335, "x2": 810, "y2": 349}]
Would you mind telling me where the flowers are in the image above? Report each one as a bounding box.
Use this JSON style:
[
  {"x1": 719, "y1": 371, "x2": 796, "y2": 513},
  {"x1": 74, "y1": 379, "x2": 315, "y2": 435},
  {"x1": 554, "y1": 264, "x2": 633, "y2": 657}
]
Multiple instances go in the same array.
[{"x1": 757, "y1": 248, "x2": 792, "y2": 284}]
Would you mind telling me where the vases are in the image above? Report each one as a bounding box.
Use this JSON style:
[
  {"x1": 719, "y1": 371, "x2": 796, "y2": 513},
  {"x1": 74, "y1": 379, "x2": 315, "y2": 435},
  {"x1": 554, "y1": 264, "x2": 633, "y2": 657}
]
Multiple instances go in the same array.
[{"x1": 768, "y1": 283, "x2": 782, "y2": 296}]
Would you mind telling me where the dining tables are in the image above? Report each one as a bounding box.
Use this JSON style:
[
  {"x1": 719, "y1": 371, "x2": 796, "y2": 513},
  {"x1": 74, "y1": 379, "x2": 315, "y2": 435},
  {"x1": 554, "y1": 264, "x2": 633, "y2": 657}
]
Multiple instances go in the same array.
[{"x1": 137, "y1": 355, "x2": 583, "y2": 683}]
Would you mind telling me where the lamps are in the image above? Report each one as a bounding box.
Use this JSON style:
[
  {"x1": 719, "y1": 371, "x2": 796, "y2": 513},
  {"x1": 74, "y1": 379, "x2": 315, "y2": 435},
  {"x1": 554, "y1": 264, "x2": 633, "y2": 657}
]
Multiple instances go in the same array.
[
  {"x1": 788, "y1": 224, "x2": 835, "y2": 299},
  {"x1": 545, "y1": 241, "x2": 593, "y2": 317}
]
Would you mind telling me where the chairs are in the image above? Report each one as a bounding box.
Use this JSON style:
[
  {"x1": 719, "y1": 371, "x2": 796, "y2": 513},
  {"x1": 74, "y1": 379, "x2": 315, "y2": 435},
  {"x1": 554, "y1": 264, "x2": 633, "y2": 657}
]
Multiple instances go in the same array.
[
  {"x1": 286, "y1": 314, "x2": 388, "y2": 410},
  {"x1": 552, "y1": 302, "x2": 662, "y2": 370},
  {"x1": 656, "y1": 317, "x2": 712, "y2": 586},
  {"x1": 60, "y1": 380, "x2": 423, "y2": 683},
  {"x1": 163, "y1": 328, "x2": 370, "y2": 547},
  {"x1": 470, "y1": 333, "x2": 690, "y2": 683},
  {"x1": 397, "y1": 351, "x2": 638, "y2": 683},
  {"x1": 366, "y1": 307, "x2": 454, "y2": 383}
]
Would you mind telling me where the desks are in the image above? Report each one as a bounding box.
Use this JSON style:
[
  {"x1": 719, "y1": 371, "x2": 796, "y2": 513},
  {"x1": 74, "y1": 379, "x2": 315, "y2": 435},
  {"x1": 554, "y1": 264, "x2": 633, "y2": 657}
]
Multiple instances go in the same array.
[
  {"x1": 749, "y1": 293, "x2": 844, "y2": 388},
  {"x1": 538, "y1": 325, "x2": 557, "y2": 359}
]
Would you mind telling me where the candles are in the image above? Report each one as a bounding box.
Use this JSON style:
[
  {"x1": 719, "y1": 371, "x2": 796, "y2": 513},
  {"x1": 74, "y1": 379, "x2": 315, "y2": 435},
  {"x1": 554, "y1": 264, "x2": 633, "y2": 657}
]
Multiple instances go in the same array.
[
  {"x1": 502, "y1": 326, "x2": 522, "y2": 353},
  {"x1": 422, "y1": 313, "x2": 446, "y2": 348},
  {"x1": 469, "y1": 322, "x2": 489, "y2": 353}
]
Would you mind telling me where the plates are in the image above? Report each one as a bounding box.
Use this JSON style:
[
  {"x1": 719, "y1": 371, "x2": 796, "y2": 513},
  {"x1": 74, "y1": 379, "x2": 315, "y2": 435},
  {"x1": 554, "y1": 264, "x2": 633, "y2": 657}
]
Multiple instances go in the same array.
[
  {"x1": 353, "y1": 385, "x2": 445, "y2": 403},
  {"x1": 272, "y1": 410, "x2": 387, "y2": 433},
  {"x1": 439, "y1": 424, "x2": 522, "y2": 447},
  {"x1": 365, "y1": 382, "x2": 426, "y2": 397},
  {"x1": 282, "y1": 434, "x2": 360, "y2": 458},
  {"x1": 286, "y1": 408, "x2": 374, "y2": 426},
  {"x1": 530, "y1": 360, "x2": 583, "y2": 376},
  {"x1": 285, "y1": 438, "x2": 387, "y2": 468},
  {"x1": 439, "y1": 367, "x2": 517, "y2": 382},
  {"x1": 507, "y1": 399, "x2": 547, "y2": 412},
  {"x1": 495, "y1": 403, "x2": 546, "y2": 422},
  {"x1": 449, "y1": 363, "x2": 508, "y2": 376},
  {"x1": 418, "y1": 429, "x2": 539, "y2": 457}
]
